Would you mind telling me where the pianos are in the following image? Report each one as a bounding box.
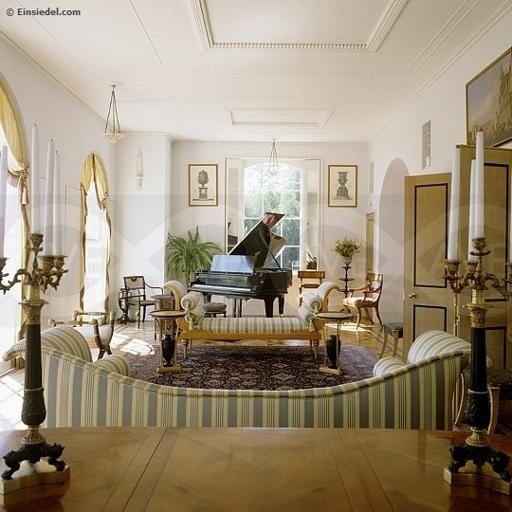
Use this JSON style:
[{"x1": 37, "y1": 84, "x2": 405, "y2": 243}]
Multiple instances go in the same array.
[{"x1": 187, "y1": 220, "x2": 292, "y2": 318}]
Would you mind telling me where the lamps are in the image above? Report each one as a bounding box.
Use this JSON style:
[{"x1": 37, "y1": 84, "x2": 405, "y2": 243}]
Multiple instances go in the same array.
[{"x1": 102, "y1": 83, "x2": 126, "y2": 144}]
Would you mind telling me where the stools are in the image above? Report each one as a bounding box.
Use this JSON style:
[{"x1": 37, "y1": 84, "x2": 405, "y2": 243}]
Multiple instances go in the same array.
[
  {"x1": 379, "y1": 321, "x2": 404, "y2": 358},
  {"x1": 203, "y1": 303, "x2": 227, "y2": 318},
  {"x1": 50, "y1": 310, "x2": 117, "y2": 360}
]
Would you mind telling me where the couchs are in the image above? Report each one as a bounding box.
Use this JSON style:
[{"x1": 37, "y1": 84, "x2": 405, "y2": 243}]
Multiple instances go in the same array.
[{"x1": 1, "y1": 326, "x2": 493, "y2": 428}]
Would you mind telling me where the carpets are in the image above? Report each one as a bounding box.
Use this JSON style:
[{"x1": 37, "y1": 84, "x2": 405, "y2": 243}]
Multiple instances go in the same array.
[{"x1": 121, "y1": 343, "x2": 378, "y2": 390}]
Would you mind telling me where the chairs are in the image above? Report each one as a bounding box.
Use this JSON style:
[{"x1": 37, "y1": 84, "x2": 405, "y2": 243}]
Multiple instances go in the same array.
[
  {"x1": 124, "y1": 275, "x2": 163, "y2": 328},
  {"x1": 343, "y1": 272, "x2": 384, "y2": 329}
]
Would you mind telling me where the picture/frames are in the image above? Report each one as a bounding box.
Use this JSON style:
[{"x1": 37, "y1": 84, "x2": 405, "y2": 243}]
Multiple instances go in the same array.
[
  {"x1": 465, "y1": 46, "x2": 512, "y2": 148},
  {"x1": 188, "y1": 163, "x2": 219, "y2": 208},
  {"x1": 327, "y1": 165, "x2": 358, "y2": 209}
]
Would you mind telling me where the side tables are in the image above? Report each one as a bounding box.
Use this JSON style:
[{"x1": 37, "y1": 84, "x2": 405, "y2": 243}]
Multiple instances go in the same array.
[
  {"x1": 149, "y1": 310, "x2": 187, "y2": 374},
  {"x1": 311, "y1": 311, "x2": 354, "y2": 375}
]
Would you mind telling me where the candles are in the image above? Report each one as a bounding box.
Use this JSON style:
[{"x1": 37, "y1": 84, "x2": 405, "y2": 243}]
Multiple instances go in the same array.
[
  {"x1": 32, "y1": 126, "x2": 62, "y2": 259},
  {"x1": 0, "y1": 145, "x2": 7, "y2": 258},
  {"x1": 447, "y1": 129, "x2": 485, "y2": 264}
]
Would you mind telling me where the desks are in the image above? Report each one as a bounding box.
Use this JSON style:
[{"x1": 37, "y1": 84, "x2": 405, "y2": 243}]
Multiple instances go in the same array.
[
  {"x1": 0, "y1": 426, "x2": 512, "y2": 511},
  {"x1": 297, "y1": 270, "x2": 326, "y2": 305}
]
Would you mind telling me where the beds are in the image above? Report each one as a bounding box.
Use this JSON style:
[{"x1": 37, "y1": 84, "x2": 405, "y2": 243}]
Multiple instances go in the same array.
[{"x1": 165, "y1": 278, "x2": 340, "y2": 362}]
